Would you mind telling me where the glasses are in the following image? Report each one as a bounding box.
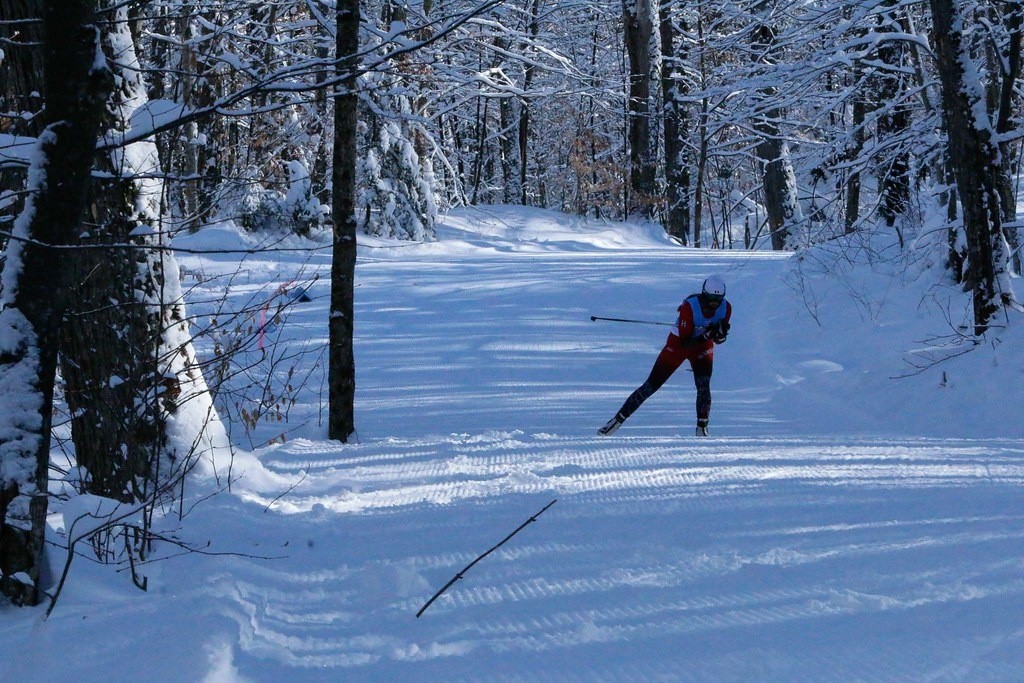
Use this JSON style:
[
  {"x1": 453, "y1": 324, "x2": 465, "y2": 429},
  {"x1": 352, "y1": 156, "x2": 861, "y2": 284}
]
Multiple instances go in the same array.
[{"x1": 706, "y1": 293, "x2": 723, "y2": 301}]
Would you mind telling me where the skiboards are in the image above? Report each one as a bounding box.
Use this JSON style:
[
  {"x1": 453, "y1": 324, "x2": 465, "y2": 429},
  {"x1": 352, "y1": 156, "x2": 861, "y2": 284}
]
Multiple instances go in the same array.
[{"x1": 595, "y1": 415, "x2": 709, "y2": 439}]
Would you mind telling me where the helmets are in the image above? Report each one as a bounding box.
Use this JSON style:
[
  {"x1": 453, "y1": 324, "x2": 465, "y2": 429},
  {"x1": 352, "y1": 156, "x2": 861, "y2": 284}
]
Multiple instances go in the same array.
[{"x1": 702, "y1": 276, "x2": 726, "y2": 296}]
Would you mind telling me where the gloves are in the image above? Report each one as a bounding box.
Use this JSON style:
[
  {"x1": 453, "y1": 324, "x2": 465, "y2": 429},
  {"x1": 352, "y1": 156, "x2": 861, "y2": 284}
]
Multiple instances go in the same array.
[{"x1": 704, "y1": 318, "x2": 730, "y2": 343}]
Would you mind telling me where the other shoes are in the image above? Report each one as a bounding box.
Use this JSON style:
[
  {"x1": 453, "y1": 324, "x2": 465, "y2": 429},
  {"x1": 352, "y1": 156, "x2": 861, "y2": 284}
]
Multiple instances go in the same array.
[
  {"x1": 698, "y1": 418, "x2": 709, "y2": 429},
  {"x1": 616, "y1": 413, "x2": 626, "y2": 424}
]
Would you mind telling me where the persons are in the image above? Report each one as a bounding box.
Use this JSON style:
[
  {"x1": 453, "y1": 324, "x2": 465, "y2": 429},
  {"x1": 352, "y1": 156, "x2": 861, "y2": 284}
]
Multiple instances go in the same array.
[{"x1": 597, "y1": 274, "x2": 732, "y2": 436}]
